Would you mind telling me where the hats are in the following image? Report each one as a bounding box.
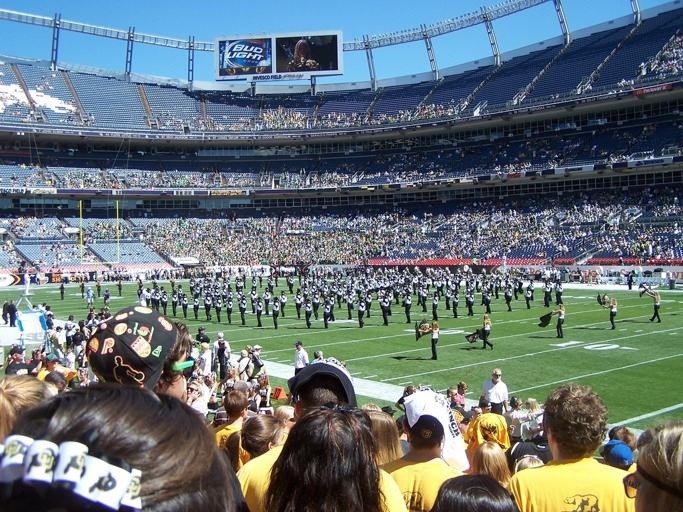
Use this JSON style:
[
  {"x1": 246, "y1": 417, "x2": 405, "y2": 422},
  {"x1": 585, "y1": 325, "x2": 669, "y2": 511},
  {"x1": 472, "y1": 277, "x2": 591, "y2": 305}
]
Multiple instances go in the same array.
[
  {"x1": 82, "y1": 305, "x2": 178, "y2": 396},
  {"x1": 599, "y1": 439, "x2": 635, "y2": 469},
  {"x1": 9, "y1": 345, "x2": 61, "y2": 364},
  {"x1": 286, "y1": 362, "x2": 359, "y2": 413},
  {"x1": 196, "y1": 326, "x2": 303, "y2": 357}
]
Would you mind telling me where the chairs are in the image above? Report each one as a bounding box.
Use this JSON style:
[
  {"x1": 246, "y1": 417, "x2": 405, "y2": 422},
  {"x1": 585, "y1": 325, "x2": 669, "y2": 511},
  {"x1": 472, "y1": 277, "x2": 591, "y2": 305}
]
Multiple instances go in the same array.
[{"x1": 0, "y1": 19, "x2": 683, "y2": 287}]
